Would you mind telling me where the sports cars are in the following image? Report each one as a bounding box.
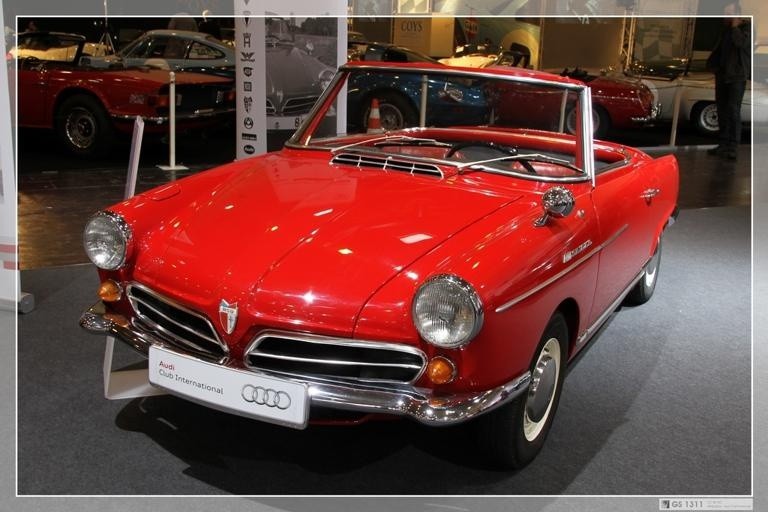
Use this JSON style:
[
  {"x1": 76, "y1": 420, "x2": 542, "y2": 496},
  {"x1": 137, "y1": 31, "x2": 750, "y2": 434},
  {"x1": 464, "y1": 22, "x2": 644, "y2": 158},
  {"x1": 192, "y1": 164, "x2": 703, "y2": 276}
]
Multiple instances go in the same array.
[
  {"x1": 77, "y1": 55, "x2": 681, "y2": 473},
  {"x1": 13, "y1": 18, "x2": 766, "y2": 154}
]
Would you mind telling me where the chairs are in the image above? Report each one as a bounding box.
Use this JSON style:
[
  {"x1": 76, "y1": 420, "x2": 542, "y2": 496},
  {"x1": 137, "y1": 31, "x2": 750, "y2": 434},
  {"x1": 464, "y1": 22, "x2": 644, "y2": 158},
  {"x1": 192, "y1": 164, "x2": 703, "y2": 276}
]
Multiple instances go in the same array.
[{"x1": 381, "y1": 141, "x2": 583, "y2": 178}]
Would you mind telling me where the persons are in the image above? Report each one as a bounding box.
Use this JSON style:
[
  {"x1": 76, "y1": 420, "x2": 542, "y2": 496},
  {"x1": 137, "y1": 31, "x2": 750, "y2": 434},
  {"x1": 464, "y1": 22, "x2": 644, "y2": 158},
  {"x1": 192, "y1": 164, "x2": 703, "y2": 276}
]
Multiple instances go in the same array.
[
  {"x1": 696, "y1": 3, "x2": 756, "y2": 161},
  {"x1": 199, "y1": 10, "x2": 223, "y2": 43},
  {"x1": 167, "y1": 1, "x2": 199, "y2": 33}
]
